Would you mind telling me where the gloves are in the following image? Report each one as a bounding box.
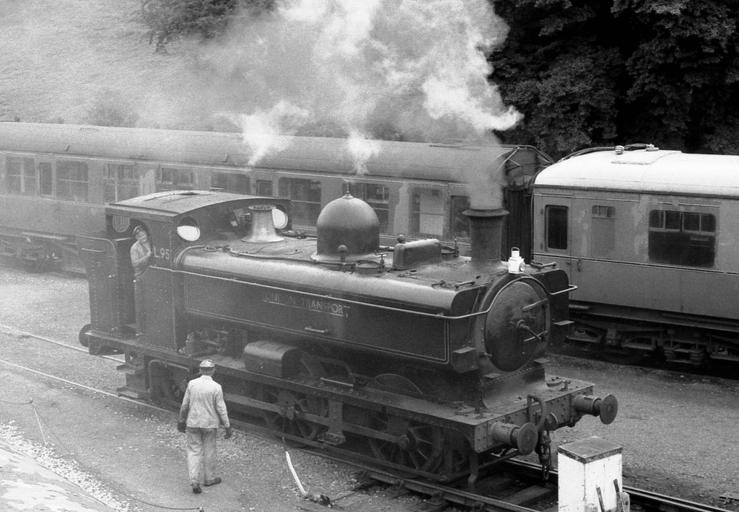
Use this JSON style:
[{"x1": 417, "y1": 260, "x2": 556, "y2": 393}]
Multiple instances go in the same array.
[
  {"x1": 223, "y1": 427, "x2": 232, "y2": 439},
  {"x1": 177, "y1": 423, "x2": 186, "y2": 432}
]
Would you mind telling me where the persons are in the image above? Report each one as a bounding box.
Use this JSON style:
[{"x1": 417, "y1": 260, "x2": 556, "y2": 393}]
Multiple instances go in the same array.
[{"x1": 177, "y1": 358, "x2": 234, "y2": 493}]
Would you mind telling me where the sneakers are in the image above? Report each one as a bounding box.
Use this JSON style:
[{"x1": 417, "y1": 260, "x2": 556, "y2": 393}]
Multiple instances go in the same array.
[
  {"x1": 204, "y1": 477, "x2": 221, "y2": 485},
  {"x1": 192, "y1": 483, "x2": 201, "y2": 493}
]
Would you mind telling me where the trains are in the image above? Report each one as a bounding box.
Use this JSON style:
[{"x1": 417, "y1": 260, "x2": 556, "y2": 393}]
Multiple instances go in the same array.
[
  {"x1": 77, "y1": 187, "x2": 619, "y2": 487},
  {"x1": 1, "y1": 121, "x2": 738, "y2": 373}
]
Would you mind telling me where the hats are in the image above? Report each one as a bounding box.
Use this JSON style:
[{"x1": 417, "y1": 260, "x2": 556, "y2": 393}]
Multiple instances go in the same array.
[
  {"x1": 132, "y1": 225, "x2": 144, "y2": 241},
  {"x1": 200, "y1": 359, "x2": 215, "y2": 368}
]
